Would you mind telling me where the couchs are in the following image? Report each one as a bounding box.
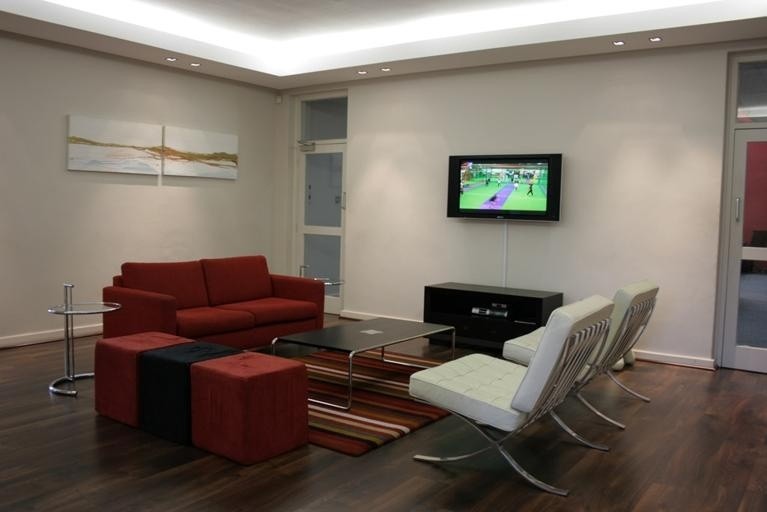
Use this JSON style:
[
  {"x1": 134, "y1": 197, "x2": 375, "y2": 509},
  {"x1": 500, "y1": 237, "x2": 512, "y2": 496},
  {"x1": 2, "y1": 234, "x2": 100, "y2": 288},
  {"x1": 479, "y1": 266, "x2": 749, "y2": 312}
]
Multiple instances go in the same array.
[{"x1": 100, "y1": 253, "x2": 325, "y2": 349}]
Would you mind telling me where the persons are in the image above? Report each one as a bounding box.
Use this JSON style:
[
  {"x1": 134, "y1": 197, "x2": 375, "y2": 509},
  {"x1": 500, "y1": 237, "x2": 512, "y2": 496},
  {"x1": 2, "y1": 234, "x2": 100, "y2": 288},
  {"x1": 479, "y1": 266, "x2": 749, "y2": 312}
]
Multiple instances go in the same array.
[{"x1": 460, "y1": 162, "x2": 536, "y2": 198}]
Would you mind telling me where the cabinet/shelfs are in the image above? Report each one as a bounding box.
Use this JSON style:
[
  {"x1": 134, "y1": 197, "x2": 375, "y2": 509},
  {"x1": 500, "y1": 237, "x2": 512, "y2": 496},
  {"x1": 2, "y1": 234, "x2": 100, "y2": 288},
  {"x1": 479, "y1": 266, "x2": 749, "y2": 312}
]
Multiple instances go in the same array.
[{"x1": 418, "y1": 279, "x2": 563, "y2": 358}]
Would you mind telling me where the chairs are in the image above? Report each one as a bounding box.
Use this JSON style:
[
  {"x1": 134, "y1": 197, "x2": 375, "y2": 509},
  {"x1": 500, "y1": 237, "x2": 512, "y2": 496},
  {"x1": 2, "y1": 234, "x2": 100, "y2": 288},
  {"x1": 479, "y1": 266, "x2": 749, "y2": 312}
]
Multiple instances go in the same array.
[
  {"x1": 503, "y1": 276, "x2": 659, "y2": 429},
  {"x1": 405, "y1": 294, "x2": 612, "y2": 497}
]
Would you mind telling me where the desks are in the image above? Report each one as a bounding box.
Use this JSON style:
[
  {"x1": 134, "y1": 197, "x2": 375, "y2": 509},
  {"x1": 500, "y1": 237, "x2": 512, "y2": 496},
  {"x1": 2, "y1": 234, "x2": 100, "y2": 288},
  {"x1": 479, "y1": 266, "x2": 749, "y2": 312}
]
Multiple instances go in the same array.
[
  {"x1": 46, "y1": 280, "x2": 122, "y2": 396},
  {"x1": 269, "y1": 316, "x2": 456, "y2": 410}
]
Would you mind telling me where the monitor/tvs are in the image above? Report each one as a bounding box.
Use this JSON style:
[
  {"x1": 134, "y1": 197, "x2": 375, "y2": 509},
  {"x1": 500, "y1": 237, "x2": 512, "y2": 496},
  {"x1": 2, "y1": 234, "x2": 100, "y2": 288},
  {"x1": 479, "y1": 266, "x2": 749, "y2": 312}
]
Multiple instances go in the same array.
[{"x1": 447, "y1": 154, "x2": 563, "y2": 220}]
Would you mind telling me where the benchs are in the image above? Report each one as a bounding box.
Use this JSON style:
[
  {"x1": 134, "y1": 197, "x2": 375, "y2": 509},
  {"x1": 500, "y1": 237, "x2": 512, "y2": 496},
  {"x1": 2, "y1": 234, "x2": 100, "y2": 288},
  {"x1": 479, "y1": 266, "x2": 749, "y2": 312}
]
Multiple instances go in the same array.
[{"x1": 92, "y1": 329, "x2": 310, "y2": 466}]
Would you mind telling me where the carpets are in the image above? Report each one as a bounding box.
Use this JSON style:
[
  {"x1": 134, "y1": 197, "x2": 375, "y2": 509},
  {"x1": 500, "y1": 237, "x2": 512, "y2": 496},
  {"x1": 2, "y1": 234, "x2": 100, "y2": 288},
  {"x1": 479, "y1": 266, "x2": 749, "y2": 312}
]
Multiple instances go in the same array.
[{"x1": 267, "y1": 349, "x2": 452, "y2": 456}]
